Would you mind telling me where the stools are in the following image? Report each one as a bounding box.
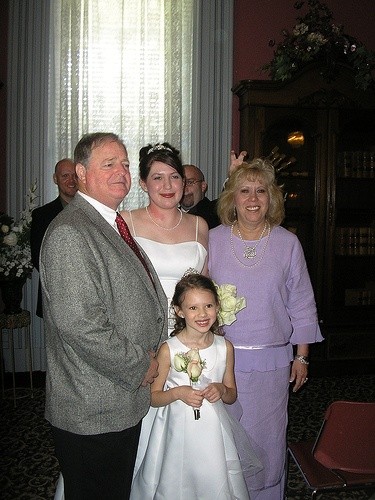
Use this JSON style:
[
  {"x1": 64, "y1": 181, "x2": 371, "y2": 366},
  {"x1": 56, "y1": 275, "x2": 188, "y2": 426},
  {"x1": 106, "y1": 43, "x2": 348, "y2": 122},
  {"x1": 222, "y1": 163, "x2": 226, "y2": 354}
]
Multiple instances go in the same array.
[{"x1": 0, "y1": 309, "x2": 36, "y2": 409}]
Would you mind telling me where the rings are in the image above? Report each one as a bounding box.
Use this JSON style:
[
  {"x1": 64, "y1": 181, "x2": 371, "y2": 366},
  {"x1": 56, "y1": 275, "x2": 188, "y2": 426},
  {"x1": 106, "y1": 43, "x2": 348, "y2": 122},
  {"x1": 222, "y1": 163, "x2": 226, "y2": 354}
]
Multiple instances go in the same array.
[{"x1": 304, "y1": 378, "x2": 308, "y2": 383}]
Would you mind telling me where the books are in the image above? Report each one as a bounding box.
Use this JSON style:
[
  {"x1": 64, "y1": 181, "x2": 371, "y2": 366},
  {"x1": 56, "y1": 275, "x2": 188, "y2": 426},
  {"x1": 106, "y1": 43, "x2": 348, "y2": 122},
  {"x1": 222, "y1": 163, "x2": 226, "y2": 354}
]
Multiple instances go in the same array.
[
  {"x1": 337, "y1": 227, "x2": 375, "y2": 256},
  {"x1": 278, "y1": 168, "x2": 309, "y2": 177},
  {"x1": 337, "y1": 150, "x2": 375, "y2": 178},
  {"x1": 344, "y1": 287, "x2": 375, "y2": 306},
  {"x1": 264, "y1": 145, "x2": 297, "y2": 174}
]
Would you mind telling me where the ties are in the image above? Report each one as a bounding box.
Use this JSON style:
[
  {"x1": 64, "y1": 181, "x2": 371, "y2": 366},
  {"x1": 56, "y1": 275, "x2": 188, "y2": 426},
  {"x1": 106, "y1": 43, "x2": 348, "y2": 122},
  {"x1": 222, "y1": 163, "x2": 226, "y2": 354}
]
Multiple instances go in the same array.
[{"x1": 115, "y1": 212, "x2": 160, "y2": 302}]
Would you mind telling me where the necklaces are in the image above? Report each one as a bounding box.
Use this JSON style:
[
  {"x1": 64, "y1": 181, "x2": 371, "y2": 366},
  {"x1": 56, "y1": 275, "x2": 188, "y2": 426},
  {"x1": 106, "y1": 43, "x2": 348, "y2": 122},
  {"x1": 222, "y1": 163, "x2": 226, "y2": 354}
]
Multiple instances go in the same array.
[
  {"x1": 231, "y1": 218, "x2": 271, "y2": 268},
  {"x1": 145, "y1": 206, "x2": 182, "y2": 231},
  {"x1": 237, "y1": 223, "x2": 267, "y2": 259}
]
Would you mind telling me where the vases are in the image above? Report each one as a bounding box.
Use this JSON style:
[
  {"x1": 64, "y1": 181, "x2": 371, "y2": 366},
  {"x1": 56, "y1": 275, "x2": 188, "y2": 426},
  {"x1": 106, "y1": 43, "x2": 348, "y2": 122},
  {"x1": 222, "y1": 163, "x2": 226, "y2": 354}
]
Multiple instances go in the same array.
[{"x1": 0, "y1": 277, "x2": 28, "y2": 314}]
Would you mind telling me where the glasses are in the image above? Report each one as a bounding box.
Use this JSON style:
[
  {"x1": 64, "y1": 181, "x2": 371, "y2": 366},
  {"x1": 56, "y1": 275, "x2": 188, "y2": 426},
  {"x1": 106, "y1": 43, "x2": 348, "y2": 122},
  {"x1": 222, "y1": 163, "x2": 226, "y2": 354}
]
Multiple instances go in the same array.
[{"x1": 186, "y1": 180, "x2": 203, "y2": 186}]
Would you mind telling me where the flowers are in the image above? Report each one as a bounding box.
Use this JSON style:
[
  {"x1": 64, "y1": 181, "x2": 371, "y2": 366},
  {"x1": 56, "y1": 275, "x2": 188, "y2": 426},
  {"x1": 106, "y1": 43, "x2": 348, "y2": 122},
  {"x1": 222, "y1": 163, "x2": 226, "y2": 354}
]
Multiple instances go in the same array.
[
  {"x1": 0, "y1": 171, "x2": 44, "y2": 280},
  {"x1": 212, "y1": 282, "x2": 248, "y2": 328},
  {"x1": 171, "y1": 347, "x2": 206, "y2": 421}
]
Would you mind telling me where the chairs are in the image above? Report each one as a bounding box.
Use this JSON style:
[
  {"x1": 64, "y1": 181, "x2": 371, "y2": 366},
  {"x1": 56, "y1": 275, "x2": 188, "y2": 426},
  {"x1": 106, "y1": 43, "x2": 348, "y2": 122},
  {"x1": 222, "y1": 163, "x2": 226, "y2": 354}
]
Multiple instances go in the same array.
[{"x1": 285, "y1": 395, "x2": 375, "y2": 500}]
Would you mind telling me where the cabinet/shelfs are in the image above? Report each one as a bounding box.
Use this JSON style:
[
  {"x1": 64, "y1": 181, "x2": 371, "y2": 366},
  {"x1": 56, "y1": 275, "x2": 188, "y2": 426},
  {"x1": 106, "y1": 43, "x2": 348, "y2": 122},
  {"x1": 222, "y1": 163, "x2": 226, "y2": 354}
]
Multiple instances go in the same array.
[{"x1": 230, "y1": 78, "x2": 375, "y2": 379}]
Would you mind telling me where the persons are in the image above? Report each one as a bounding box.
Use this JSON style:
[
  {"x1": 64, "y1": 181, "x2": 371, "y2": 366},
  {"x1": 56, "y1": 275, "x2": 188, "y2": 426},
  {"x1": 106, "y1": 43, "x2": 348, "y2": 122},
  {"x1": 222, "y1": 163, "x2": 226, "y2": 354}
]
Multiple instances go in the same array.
[
  {"x1": 206, "y1": 160, "x2": 324, "y2": 500},
  {"x1": 129, "y1": 275, "x2": 250, "y2": 500},
  {"x1": 31, "y1": 159, "x2": 79, "y2": 319},
  {"x1": 40, "y1": 133, "x2": 169, "y2": 500},
  {"x1": 179, "y1": 151, "x2": 248, "y2": 229},
  {"x1": 51, "y1": 143, "x2": 210, "y2": 500}
]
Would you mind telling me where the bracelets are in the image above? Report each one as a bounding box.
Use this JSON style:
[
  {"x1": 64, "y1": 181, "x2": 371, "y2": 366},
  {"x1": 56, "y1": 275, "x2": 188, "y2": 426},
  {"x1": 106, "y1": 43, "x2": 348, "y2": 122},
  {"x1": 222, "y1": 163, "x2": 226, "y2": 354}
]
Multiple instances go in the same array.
[{"x1": 295, "y1": 355, "x2": 309, "y2": 364}]
